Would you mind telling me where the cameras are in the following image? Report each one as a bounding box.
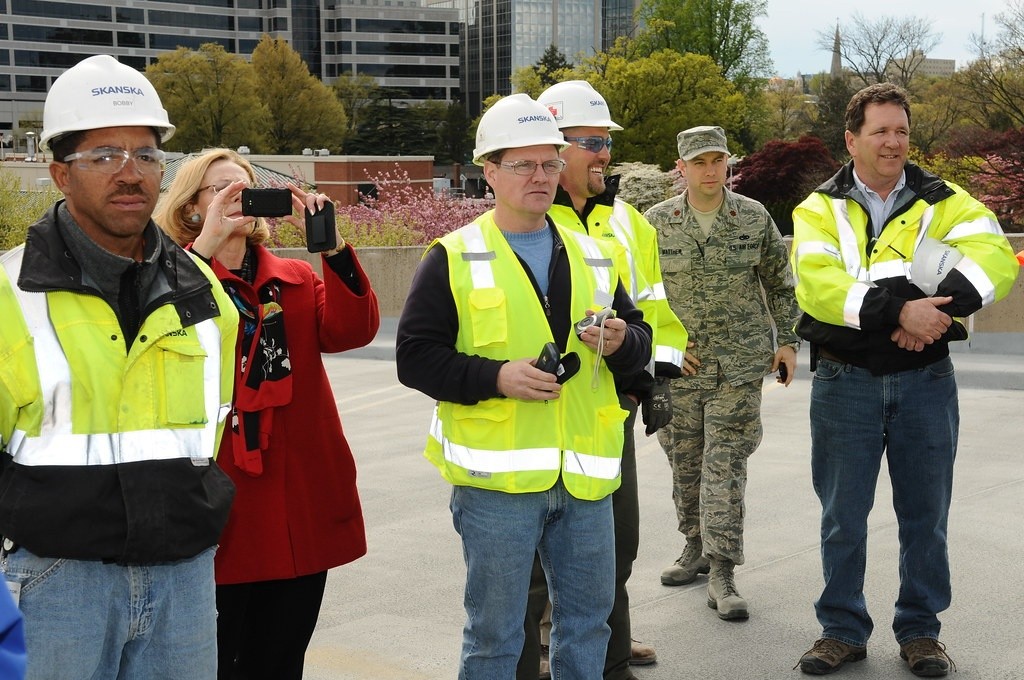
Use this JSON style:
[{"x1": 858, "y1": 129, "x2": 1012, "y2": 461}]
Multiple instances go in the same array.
[{"x1": 573, "y1": 307, "x2": 615, "y2": 341}]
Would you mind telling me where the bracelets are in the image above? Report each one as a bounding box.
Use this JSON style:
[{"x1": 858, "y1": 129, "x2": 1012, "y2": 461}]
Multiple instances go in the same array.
[{"x1": 321, "y1": 239, "x2": 346, "y2": 257}]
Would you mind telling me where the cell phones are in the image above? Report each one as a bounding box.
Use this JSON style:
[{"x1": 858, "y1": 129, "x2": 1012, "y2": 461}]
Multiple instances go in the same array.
[
  {"x1": 242, "y1": 188, "x2": 292, "y2": 217},
  {"x1": 778, "y1": 362, "x2": 788, "y2": 383}
]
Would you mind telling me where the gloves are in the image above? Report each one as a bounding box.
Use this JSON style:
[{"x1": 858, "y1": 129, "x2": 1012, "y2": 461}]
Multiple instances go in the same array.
[{"x1": 642, "y1": 376, "x2": 673, "y2": 437}]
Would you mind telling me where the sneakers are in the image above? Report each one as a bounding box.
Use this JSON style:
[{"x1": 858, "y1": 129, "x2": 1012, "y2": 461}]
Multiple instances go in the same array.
[
  {"x1": 627, "y1": 637, "x2": 656, "y2": 663},
  {"x1": 792, "y1": 638, "x2": 868, "y2": 676},
  {"x1": 538, "y1": 642, "x2": 550, "y2": 680},
  {"x1": 899, "y1": 638, "x2": 958, "y2": 677}
]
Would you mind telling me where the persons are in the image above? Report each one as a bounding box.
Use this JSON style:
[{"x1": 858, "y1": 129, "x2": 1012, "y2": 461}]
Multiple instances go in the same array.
[
  {"x1": 641, "y1": 125, "x2": 803, "y2": 622},
  {"x1": 537, "y1": 80, "x2": 690, "y2": 680},
  {"x1": 395, "y1": 92, "x2": 653, "y2": 680},
  {"x1": 147, "y1": 148, "x2": 379, "y2": 680},
  {"x1": 0, "y1": 53, "x2": 242, "y2": 680},
  {"x1": 790, "y1": 83, "x2": 1020, "y2": 677}
]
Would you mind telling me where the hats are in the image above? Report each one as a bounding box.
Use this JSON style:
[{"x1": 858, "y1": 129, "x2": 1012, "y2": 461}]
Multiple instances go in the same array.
[{"x1": 676, "y1": 126, "x2": 732, "y2": 161}]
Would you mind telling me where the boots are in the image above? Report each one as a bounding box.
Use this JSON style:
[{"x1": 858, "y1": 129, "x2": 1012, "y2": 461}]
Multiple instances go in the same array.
[
  {"x1": 661, "y1": 535, "x2": 710, "y2": 586},
  {"x1": 706, "y1": 556, "x2": 750, "y2": 618}
]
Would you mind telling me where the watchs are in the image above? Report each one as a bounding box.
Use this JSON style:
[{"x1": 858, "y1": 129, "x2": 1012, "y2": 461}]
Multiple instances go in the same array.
[{"x1": 787, "y1": 342, "x2": 798, "y2": 354}]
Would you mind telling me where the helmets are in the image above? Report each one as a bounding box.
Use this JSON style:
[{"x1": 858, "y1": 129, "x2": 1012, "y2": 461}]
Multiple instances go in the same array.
[
  {"x1": 39, "y1": 55, "x2": 175, "y2": 151},
  {"x1": 911, "y1": 238, "x2": 961, "y2": 297},
  {"x1": 536, "y1": 80, "x2": 624, "y2": 131},
  {"x1": 472, "y1": 94, "x2": 572, "y2": 166}
]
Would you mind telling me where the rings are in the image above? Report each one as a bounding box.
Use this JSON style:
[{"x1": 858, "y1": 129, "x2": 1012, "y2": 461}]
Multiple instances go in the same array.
[{"x1": 606, "y1": 339, "x2": 609, "y2": 348}]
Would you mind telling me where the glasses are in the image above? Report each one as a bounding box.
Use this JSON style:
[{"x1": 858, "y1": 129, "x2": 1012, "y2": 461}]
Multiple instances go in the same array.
[
  {"x1": 61, "y1": 145, "x2": 167, "y2": 174},
  {"x1": 564, "y1": 135, "x2": 613, "y2": 155},
  {"x1": 190, "y1": 182, "x2": 258, "y2": 196},
  {"x1": 493, "y1": 158, "x2": 566, "y2": 175}
]
